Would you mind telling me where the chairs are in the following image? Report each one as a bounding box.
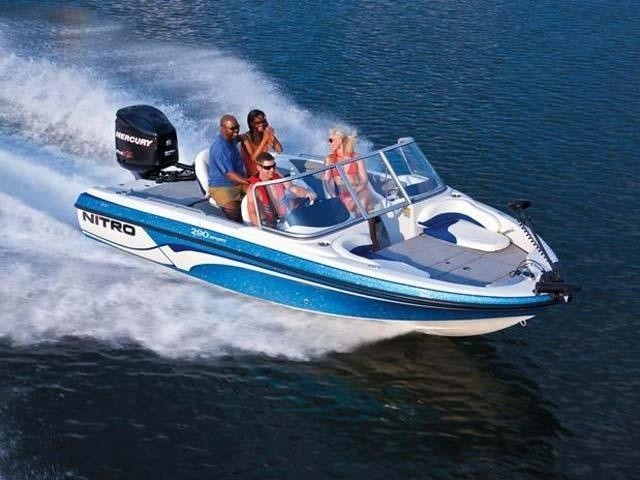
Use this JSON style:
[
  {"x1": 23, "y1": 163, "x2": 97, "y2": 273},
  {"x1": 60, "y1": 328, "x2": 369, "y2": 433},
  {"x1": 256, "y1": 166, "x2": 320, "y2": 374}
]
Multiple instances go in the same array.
[
  {"x1": 241, "y1": 193, "x2": 266, "y2": 228},
  {"x1": 193, "y1": 148, "x2": 227, "y2": 210}
]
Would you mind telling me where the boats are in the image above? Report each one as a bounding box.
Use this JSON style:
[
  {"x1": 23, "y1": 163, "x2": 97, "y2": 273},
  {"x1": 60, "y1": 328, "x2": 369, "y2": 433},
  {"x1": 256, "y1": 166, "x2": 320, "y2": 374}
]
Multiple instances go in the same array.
[{"x1": 74, "y1": 103, "x2": 585, "y2": 338}]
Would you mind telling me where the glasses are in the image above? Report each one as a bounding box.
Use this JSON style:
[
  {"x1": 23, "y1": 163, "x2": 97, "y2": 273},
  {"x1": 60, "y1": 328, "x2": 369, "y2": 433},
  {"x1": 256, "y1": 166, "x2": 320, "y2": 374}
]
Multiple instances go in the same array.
[
  {"x1": 223, "y1": 125, "x2": 240, "y2": 130},
  {"x1": 259, "y1": 163, "x2": 276, "y2": 170},
  {"x1": 329, "y1": 138, "x2": 335, "y2": 143}
]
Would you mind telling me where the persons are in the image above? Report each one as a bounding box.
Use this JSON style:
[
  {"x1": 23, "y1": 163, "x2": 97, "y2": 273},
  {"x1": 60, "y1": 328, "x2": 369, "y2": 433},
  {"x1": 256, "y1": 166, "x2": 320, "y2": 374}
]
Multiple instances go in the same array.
[
  {"x1": 323, "y1": 128, "x2": 372, "y2": 218},
  {"x1": 206, "y1": 114, "x2": 246, "y2": 223},
  {"x1": 240, "y1": 109, "x2": 283, "y2": 177},
  {"x1": 243, "y1": 152, "x2": 317, "y2": 230}
]
